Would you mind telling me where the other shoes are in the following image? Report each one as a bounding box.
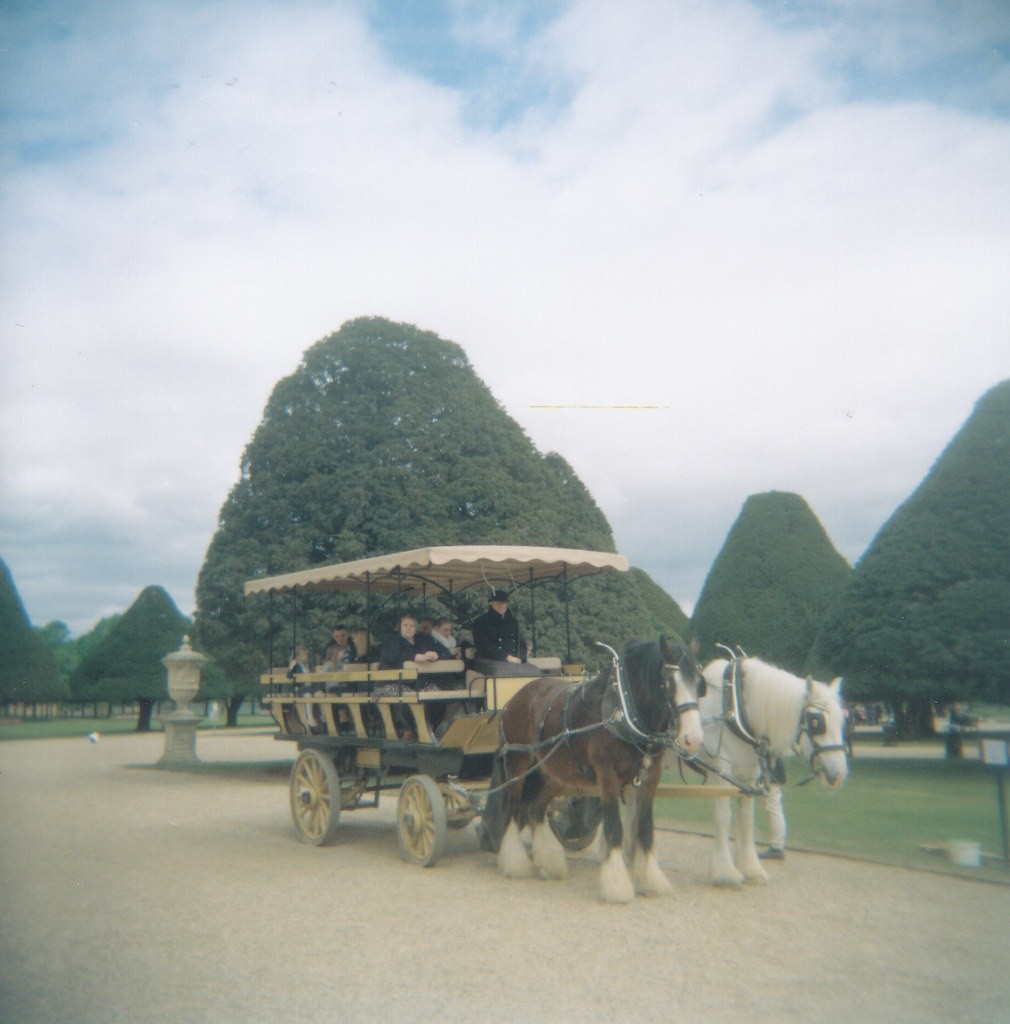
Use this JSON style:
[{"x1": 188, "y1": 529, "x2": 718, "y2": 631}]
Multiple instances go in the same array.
[
  {"x1": 308, "y1": 722, "x2": 321, "y2": 734},
  {"x1": 400, "y1": 727, "x2": 413, "y2": 742}
]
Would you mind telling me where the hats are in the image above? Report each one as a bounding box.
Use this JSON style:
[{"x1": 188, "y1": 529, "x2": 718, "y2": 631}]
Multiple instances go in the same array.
[{"x1": 487, "y1": 590, "x2": 510, "y2": 603}]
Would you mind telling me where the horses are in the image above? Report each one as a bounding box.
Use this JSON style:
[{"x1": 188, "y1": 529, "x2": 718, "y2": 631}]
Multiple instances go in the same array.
[
  {"x1": 599, "y1": 657, "x2": 848, "y2": 888},
  {"x1": 496, "y1": 632, "x2": 703, "y2": 902}
]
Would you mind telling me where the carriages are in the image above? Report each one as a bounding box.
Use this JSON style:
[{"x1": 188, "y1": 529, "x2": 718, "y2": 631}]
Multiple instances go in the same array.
[{"x1": 242, "y1": 546, "x2": 851, "y2": 904}]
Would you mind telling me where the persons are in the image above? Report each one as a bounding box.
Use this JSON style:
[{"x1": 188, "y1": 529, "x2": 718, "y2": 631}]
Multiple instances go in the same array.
[
  {"x1": 474, "y1": 591, "x2": 544, "y2": 677},
  {"x1": 841, "y1": 694, "x2": 979, "y2": 762},
  {"x1": 289, "y1": 614, "x2": 474, "y2": 744}
]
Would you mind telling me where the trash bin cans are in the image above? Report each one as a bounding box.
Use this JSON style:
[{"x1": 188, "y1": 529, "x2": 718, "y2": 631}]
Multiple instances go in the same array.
[{"x1": 882, "y1": 718, "x2": 900, "y2": 747}]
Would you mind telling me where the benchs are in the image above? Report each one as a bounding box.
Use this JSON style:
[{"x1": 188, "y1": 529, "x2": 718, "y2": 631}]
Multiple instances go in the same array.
[{"x1": 265, "y1": 656, "x2": 562, "y2": 745}]
